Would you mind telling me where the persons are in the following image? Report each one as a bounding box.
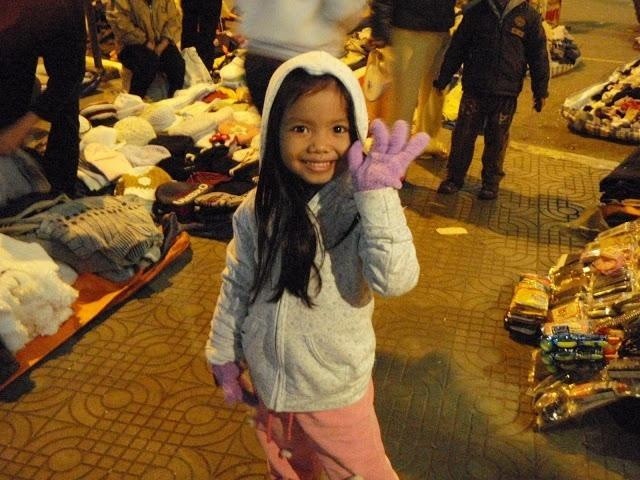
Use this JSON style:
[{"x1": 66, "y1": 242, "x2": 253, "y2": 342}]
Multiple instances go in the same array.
[
  {"x1": 370, "y1": 2, "x2": 458, "y2": 174},
  {"x1": 430, "y1": 0, "x2": 551, "y2": 201},
  {"x1": 232, "y1": 0, "x2": 371, "y2": 123},
  {"x1": 178, "y1": 0, "x2": 224, "y2": 78},
  {"x1": 103, "y1": 0, "x2": 186, "y2": 99},
  {"x1": 0, "y1": 0, "x2": 87, "y2": 196},
  {"x1": 199, "y1": 49, "x2": 435, "y2": 480}
]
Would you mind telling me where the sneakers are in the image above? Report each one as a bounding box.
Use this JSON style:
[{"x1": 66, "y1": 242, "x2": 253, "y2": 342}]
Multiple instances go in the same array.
[
  {"x1": 438, "y1": 179, "x2": 463, "y2": 193},
  {"x1": 416, "y1": 148, "x2": 450, "y2": 160},
  {"x1": 479, "y1": 185, "x2": 499, "y2": 199}
]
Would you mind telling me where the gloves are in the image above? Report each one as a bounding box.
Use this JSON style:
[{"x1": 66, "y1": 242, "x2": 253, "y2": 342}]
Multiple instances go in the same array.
[
  {"x1": 348, "y1": 119, "x2": 430, "y2": 192},
  {"x1": 211, "y1": 361, "x2": 258, "y2": 407}
]
